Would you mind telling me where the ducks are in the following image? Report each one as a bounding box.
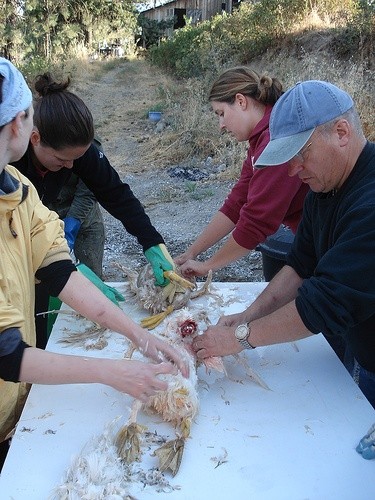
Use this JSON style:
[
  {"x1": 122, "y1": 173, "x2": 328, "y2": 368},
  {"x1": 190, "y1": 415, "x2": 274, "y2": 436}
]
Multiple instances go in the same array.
[
  {"x1": 117, "y1": 262, "x2": 213, "y2": 331},
  {"x1": 111, "y1": 308, "x2": 271, "y2": 479}
]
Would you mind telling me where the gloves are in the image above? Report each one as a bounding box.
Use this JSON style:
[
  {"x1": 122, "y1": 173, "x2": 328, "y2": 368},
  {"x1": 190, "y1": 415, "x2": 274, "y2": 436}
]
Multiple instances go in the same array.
[
  {"x1": 143, "y1": 244, "x2": 173, "y2": 287},
  {"x1": 77, "y1": 263, "x2": 125, "y2": 310},
  {"x1": 356, "y1": 422, "x2": 375, "y2": 460},
  {"x1": 64, "y1": 217, "x2": 81, "y2": 252}
]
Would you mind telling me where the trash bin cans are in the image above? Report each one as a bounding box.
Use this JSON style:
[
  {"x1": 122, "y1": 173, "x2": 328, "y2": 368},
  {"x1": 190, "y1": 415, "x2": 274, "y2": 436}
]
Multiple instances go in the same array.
[{"x1": 255, "y1": 227, "x2": 296, "y2": 283}]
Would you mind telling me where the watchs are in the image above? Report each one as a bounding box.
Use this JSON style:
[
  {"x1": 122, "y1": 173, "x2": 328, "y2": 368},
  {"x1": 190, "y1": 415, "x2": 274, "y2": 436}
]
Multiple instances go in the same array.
[{"x1": 234, "y1": 321, "x2": 255, "y2": 350}]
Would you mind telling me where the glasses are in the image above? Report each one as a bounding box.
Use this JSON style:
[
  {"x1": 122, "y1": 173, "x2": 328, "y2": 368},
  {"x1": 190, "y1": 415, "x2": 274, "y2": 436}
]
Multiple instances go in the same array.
[{"x1": 288, "y1": 122, "x2": 336, "y2": 163}]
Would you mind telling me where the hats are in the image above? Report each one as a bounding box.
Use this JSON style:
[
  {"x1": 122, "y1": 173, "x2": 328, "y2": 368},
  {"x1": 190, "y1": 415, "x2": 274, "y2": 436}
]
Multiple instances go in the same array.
[{"x1": 253, "y1": 80, "x2": 354, "y2": 169}]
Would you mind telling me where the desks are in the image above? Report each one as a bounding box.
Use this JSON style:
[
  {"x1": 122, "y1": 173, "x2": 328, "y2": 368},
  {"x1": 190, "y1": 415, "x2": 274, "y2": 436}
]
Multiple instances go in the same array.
[{"x1": 0, "y1": 281, "x2": 375, "y2": 500}]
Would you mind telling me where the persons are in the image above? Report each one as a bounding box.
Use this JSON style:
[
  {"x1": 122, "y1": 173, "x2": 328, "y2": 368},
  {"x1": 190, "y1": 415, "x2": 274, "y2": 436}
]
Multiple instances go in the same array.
[
  {"x1": 1, "y1": 59, "x2": 187, "y2": 473},
  {"x1": 190, "y1": 80, "x2": 375, "y2": 410},
  {"x1": 170, "y1": 65, "x2": 311, "y2": 282},
  {"x1": 10, "y1": 71, "x2": 175, "y2": 350},
  {"x1": 56, "y1": 136, "x2": 104, "y2": 280}
]
